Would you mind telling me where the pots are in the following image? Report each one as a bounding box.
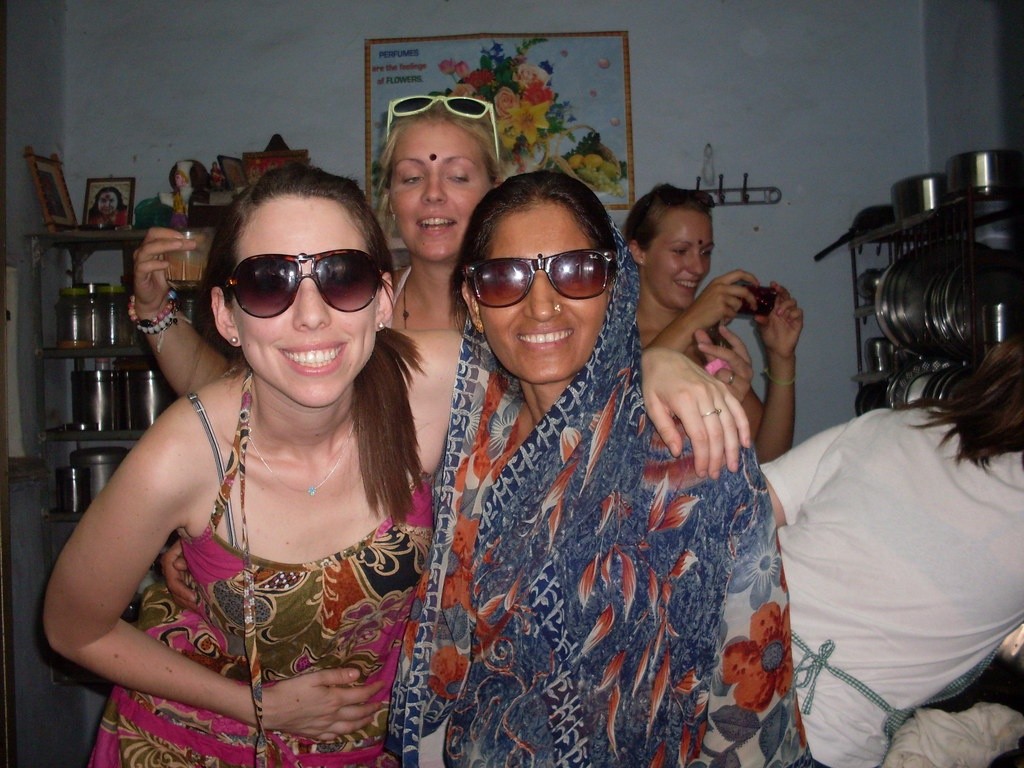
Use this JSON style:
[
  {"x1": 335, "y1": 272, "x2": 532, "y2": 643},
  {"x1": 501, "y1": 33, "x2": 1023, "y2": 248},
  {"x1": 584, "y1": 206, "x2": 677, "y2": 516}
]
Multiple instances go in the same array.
[
  {"x1": 852, "y1": 214, "x2": 1024, "y2": 357},
  {"x1": 813, "y1": 203, "x2": 894, "y2": 261}
]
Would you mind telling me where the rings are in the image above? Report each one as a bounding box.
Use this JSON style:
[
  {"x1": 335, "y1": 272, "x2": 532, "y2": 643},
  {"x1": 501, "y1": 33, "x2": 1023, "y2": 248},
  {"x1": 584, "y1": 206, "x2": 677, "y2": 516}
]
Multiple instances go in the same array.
[
  {"x1": 728, "y1": 372, "x2": 736, "y2": 386},
  {"x1": 701, "y1": 407, "x2": 721, "y2": 417}
]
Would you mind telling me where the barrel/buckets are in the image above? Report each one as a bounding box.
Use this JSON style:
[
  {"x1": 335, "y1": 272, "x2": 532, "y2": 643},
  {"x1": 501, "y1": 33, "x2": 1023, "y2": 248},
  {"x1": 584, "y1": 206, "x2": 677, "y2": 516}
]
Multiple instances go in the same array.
[{"x1": 54, "y1": 367, "x2": 170, "y2": 514}]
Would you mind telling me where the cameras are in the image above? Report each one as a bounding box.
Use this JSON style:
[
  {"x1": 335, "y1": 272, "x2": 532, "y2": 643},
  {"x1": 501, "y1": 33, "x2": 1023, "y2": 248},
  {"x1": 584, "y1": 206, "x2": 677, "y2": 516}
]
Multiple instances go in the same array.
[{"x1": 738, "y1": 285, "x2": 778, "y2": 317}]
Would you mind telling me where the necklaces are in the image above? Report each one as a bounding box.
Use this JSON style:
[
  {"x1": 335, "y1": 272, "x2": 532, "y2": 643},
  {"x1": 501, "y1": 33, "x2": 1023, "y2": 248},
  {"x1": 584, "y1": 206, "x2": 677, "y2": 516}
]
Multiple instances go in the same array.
[
  {"x1": 241, "y1": 419, "x2": 357, "y2": 497},
  {"x1": 402, "y1": 281, "x2": 409, "y2": 330}
]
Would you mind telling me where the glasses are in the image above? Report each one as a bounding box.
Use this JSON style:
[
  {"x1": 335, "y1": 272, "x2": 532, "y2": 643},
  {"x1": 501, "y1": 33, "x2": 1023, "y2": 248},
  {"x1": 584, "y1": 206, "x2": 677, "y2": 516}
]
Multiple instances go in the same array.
[
  {"x1": 223, "y1": 247, "x2": 389, "y2": 318},
  {"x1": 387, "y1": 95, "x2": 499, "y2": 167},
  {"x1": 631, "y1": 184, "x2": 716, "y2": 242},
  {"x1": 466, "y1": 249, "x2": 619, "y2": 308}
]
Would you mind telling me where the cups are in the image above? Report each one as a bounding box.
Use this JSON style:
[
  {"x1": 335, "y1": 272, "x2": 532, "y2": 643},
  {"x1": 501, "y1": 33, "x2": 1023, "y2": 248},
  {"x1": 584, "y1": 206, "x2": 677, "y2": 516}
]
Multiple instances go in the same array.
[
  {"x1": 863, "y1": 335, "x2": 894, "y2": 373},
  {"x1": 160, "y1": 227, "x2": 218, "y2": 290}
]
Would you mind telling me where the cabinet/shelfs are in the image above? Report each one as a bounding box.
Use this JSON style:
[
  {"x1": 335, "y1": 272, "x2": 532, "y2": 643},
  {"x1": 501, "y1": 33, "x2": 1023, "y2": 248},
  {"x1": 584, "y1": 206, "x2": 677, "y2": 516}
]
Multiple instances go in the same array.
[
  {"x1": 30, "y1": 228, "x2": 244, "y2": 570},
  {"x1": 847, "y1": 182, "x2": 1024, "y2": 392}
]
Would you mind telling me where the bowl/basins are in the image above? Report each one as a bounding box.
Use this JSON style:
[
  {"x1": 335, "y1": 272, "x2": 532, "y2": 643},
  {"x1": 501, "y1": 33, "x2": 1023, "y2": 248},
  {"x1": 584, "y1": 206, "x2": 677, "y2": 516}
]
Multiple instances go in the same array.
[
  {"x1": 946, "y1": 149, "x2": 1024, "y2": 194},
  {"x1": 890, "y1": 173, "x2": 949, "y2": 224}
]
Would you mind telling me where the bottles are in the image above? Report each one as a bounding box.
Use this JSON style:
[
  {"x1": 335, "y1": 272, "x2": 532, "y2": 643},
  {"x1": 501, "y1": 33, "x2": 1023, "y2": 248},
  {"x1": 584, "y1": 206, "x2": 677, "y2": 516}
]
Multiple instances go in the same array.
[
  {"x1": 94, "y1": 357, "x2": 111, "y2": 371},
  {"x1": 53, "y1": 282, "x2": 134, "y2": 349}
]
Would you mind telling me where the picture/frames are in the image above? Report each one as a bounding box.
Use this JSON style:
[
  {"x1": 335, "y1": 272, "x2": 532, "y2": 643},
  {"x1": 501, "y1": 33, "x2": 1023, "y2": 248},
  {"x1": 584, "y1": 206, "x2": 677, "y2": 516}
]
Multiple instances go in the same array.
[
  {"x1": 81, "y1": 176, "x2": 136, "y2": 231},
  {"x1": 22, "y1": 145, "x2": 82, "y2": 234}
]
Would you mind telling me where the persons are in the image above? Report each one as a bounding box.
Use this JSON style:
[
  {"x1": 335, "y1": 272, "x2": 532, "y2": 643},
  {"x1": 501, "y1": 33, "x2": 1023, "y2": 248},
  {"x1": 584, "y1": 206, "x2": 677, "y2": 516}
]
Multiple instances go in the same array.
[
  {"x1": 124, "y1": 93, "x2": 756, "y2": 403},
  {"x1": 622, "y1": 184, "x2": 804, "y2": 465},
  {"x1": 380, "y1": 170, "x2": 819, "y2": 768},
  {"x1": 88, "y1": 188, "x2": 129, "y2": 224},
  {"x1": 160, "y1": 161, "x2": 193, "y2": 227},
  {"x1": 754, "y1": 333, "x2": 1024, "y2": 767},
  {"x1": 42, "y1": 167, "x2": 752, "y2": 768}
]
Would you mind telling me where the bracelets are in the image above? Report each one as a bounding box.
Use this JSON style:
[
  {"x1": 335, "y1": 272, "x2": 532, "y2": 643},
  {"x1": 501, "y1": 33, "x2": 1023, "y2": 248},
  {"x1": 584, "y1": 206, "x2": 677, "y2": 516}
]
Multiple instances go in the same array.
[
  {"x1": 763, "y1": 367, "x2": 796, "y2": 385},
  {"x1": 128, "y1": 288, "x2": 182, "y2": 334}
]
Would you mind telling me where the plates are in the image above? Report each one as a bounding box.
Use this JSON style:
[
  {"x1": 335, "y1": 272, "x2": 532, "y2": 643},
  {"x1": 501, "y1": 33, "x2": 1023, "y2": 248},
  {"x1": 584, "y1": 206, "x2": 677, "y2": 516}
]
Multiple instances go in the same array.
[{"x1": 854, "y1": 360, "x2": 978, "y2": 418}]
